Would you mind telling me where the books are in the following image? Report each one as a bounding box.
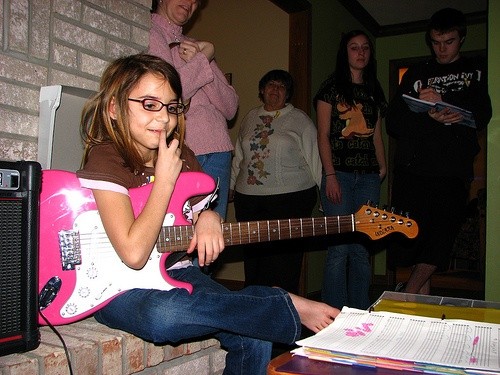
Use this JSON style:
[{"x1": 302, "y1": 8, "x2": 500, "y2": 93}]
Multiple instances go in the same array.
[{"x1": 402, "y1": 93, "x2": 476, "y2": 128}]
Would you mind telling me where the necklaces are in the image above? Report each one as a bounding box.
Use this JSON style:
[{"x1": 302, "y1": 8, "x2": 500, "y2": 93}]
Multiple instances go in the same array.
[{"x1": 167, "y1": 20, "x2": 182, "y2": 42}]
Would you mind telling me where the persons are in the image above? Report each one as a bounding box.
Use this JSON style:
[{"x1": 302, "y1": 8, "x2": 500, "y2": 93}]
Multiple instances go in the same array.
[
  {"x1": 77, "y1": 55, "x2": 340, "y2": 375},
  {"x1": 150, "y1": 0, "x2": 240, "y2": 267},
  {"x1": 313, "y1": 30, "x2": 387, "y2": 312},
  {"x1": 227, "y1": 70, "x2": 322, "y2": 296},
  {"x1": 384, "y1": 9, "x2": 492, "y2": 296}
]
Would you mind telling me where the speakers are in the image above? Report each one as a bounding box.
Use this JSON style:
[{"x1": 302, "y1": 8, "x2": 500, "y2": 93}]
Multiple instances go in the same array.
[{"x1": 0, "y1": 159, "x2": 42, "y2": 357}]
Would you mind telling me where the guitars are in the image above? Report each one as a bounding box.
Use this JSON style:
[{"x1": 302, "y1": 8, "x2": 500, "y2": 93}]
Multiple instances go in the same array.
[{"x1": 38, "y1": 169, "x2": 418, "y2": 326}]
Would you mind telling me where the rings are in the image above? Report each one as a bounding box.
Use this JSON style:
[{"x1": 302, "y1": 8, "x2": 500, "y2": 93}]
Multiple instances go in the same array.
[{"x1": 183, "y1": 49, "x2": 186, "y2": 54}]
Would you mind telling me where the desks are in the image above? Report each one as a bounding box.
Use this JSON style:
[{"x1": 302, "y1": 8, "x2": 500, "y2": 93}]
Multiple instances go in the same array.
[{"x1": 266, "y1": 348, "x2": 426, "y2": 375}]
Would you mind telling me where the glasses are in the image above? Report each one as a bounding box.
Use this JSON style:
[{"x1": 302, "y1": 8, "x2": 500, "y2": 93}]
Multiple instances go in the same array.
[{"x1": 128, "y1": 98, "x2": 186, "y2": 114}]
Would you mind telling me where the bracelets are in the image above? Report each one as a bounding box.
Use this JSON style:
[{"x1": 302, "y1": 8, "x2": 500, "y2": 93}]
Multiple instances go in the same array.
[{"x1": 326, "y1": 174, "x2": 336, "y2": 176}]
